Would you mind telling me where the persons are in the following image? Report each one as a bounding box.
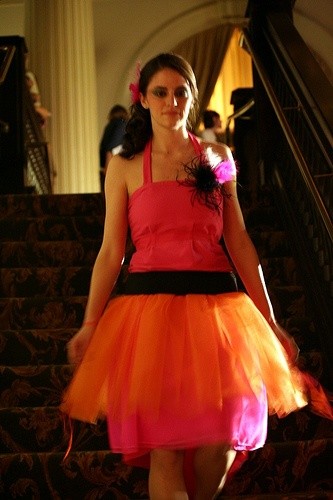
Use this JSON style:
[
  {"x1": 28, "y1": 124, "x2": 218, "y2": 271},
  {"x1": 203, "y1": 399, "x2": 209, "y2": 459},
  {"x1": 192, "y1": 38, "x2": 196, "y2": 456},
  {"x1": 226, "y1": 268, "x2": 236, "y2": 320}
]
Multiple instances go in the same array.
[
  {"x1": 201, "y1": 108, "x2": 222, "y2": 142},
  {"x1": 57, "y1": 53, "x2": 309, "y2": 500},
  {"x1": 25, "y1": 55, "x2": 54, "y2": 193},
  {"x1": 100, "y1": 105, "x2": 129, "y2": 192}
]
